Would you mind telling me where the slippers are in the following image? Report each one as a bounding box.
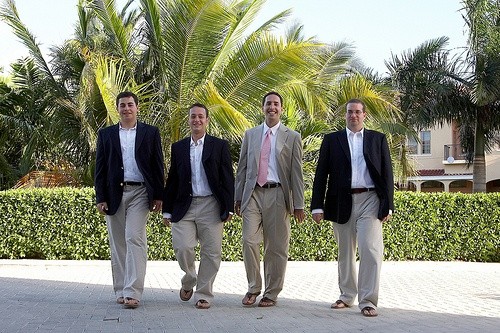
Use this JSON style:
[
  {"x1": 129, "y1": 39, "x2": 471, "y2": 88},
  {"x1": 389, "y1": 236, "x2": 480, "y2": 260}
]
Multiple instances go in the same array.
[
  {"x1": 331, "y1": 299, "x2": 349, "y2": 308},
  {"x1": 180, "y1": 288, "x2": 193, "y2": 301},
  {"x1": 195, "y1": 299, "x2": 210, "y2": 309},
  {"x1": 116, "y1": 297, "x2": 124, "y2": 304},
  {"x1": 124, "y1": 297, "x2": 139, "y2": 308},
  {"x1": 242, "y1": 292, "x2": 261, "y2": 305},
  {"x1": 257, "y1": 297, "x2": 278, "y2": 307},
  {"x1": 361, "y1": 305, "x2": 378, "y2": 316}
]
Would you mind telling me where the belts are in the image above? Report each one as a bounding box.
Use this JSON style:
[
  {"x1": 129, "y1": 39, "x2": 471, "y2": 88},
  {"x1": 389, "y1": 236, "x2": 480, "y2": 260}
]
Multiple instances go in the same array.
[
  {"x1": 255, "y1": 181, "x2": 281, "y2": 189},
  {"x1": 192, "y1": 193, "x2": 215, "y2": 198},
  {"x1": 117, "y1": 181, "x2": 147, "y2": 187},
  {"x1": 350, "y1": 187, "x2": 377, "y2": 194}
]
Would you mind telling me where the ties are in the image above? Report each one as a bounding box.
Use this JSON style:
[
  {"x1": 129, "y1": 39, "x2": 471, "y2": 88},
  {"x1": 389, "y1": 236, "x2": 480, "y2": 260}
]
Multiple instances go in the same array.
[{"x1": 257, "y1": 128, "x2": 272, "y2": 187}]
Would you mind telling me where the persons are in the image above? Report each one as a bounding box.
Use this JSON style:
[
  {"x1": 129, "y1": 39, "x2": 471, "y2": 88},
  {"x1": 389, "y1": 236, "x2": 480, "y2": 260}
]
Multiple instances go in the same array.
[
  {"x1": 235, "y1": 92, "x2": 306, "y2": 307},
  {"x1": 162, "y1": 103, "x2": 235, "y2": 309},
  {"x1": 95, "y1": 92, "x2": 165, "y2": 307},
  {"x1": 310, "y1": 98, "x2": 394, "y2": 317}
]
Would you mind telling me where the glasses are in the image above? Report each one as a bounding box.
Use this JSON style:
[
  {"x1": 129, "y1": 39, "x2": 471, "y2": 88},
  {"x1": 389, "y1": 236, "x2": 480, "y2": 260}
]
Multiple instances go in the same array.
[{"x1": 346, "y1": 109, "x2": 364, "y2": 115}]
